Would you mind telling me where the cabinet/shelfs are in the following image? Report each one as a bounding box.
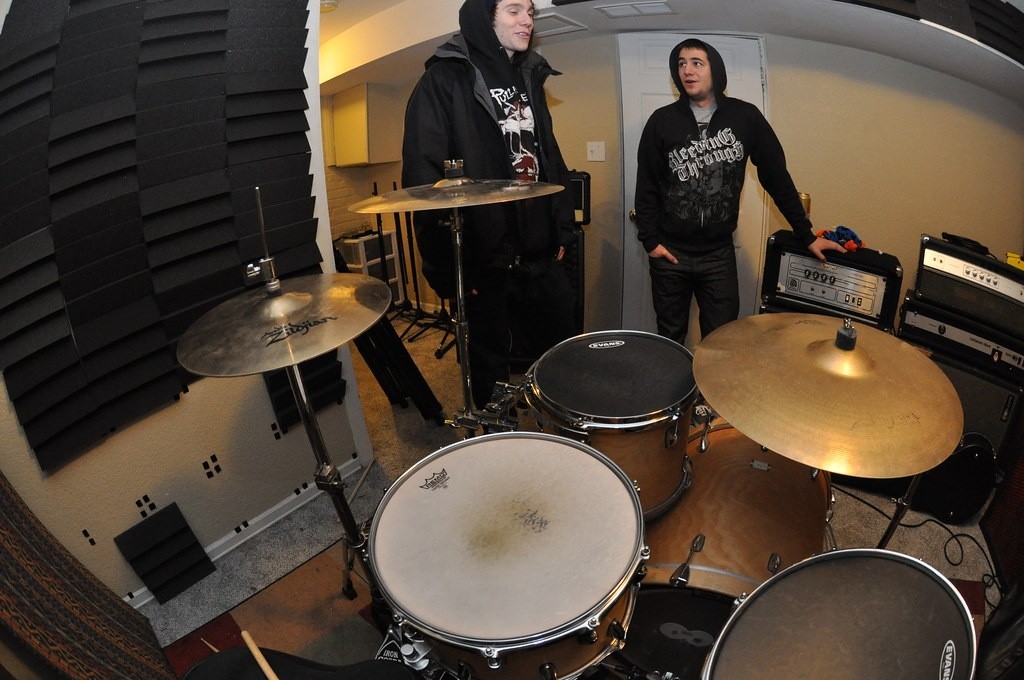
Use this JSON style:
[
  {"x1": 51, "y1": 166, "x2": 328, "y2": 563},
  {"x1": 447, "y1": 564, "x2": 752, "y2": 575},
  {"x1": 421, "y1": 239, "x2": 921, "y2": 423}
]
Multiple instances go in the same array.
[
  {"x1": 330, "y1": 82, "x2": 400, "y2": 168},
  {"x1": 335, "y1": 230, "x2": 405, "y2": 307}
]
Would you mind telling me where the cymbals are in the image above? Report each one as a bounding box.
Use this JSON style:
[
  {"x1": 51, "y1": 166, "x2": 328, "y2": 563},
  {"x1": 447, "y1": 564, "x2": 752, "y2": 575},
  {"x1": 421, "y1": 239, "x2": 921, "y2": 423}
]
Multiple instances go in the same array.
[
  {"x1": 347, "y1": 177, "x2": 564, "y2": 214},
  {"x1": 693, "y1": 311, "x2": 964, "y2": 477},
  {"x1": 177, "y1": 272, "x2": 392, "y2": 379}
]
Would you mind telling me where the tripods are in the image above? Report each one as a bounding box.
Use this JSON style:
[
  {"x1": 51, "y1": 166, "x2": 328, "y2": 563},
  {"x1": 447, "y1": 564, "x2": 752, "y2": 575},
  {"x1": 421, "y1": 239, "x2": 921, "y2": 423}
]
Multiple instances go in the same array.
[{"x1": 286, "y1": 364, "x2": 390, "y2": 610}]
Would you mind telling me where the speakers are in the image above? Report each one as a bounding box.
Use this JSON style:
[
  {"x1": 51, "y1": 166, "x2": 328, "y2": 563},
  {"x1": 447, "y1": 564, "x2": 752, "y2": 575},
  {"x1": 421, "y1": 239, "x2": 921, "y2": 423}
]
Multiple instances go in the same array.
[{"x1": 830, "y1": 347, "x2": 1024, "y2": 527}]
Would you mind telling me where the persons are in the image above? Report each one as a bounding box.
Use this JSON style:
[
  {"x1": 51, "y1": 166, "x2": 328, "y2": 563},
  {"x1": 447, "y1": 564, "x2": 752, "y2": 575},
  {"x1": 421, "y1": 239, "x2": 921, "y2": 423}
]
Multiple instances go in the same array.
[
  {"x1": 400, "y1": 1, "x2": 578, "y2": 410},
  {"x1": 634, "y1": 38, "x2": 846, "y2": 348}
]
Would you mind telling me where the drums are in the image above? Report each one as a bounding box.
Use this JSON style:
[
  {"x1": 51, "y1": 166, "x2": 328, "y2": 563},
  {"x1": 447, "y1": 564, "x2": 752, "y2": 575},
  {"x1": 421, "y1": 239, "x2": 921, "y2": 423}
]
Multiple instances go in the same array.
[
  {"x1": 697, "y1": 546, "x2": 977, "y2": 680},
  {"x1": 520, "y1": 330, "x2": 699, "y2": 520},
  {"x1": 367, "y1": 429, "x2": 650, "y2": 680},
  {"x1": 617, "y1": 413, "x2": 832, "y2": 680}
]
